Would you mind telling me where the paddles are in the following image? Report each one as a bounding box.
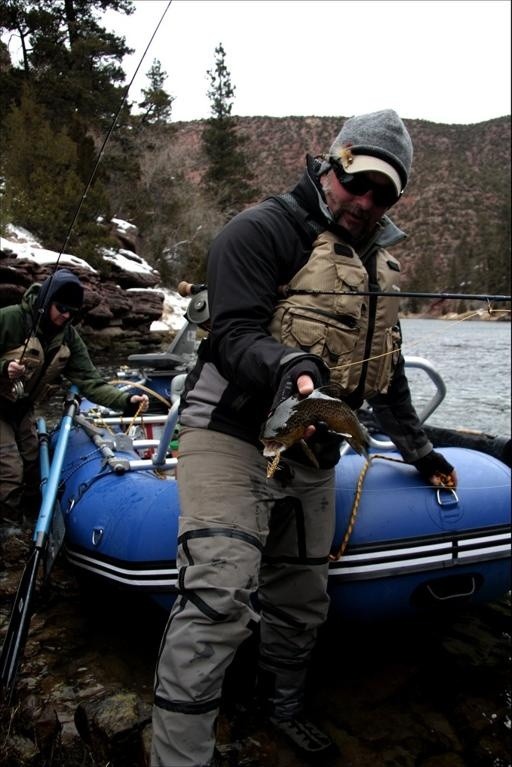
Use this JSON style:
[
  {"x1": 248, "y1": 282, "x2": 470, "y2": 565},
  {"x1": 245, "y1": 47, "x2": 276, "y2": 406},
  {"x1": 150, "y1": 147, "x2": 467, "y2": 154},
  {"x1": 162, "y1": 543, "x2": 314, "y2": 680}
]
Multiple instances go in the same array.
[{"x1": 0, "y1": 383, "x2": 81, "y2": 694}]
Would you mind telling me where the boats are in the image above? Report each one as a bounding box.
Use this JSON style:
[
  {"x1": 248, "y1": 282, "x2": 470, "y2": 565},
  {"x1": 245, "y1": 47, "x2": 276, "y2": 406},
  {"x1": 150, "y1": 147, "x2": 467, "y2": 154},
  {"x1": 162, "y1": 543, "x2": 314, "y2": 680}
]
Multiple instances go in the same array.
[{"x1": 44, "y1": 362, "x2": 510, "y2": 664}]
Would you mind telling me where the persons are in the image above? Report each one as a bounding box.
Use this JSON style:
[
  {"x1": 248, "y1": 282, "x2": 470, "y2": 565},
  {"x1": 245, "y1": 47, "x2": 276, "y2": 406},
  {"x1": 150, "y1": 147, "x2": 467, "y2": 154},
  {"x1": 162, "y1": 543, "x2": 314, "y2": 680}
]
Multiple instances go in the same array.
[
  {"x1": 150, "y1": 110, "x2": 467, "y2": 764},
  {"x1": 0, "y1": 268, "x2": 149, "y2": 522}
]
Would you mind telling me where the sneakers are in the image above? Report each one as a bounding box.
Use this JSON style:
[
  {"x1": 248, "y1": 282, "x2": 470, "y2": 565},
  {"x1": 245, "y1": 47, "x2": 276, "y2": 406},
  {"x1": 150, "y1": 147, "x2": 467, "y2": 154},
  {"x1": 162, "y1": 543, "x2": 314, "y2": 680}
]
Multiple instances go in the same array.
[{"x1": 261, "y1": 712, "x2": 343, "y2": 767}]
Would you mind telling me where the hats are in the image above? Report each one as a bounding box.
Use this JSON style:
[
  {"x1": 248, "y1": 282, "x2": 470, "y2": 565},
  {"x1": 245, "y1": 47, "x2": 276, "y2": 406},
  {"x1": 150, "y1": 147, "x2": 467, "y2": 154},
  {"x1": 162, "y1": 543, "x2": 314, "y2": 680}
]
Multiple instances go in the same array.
[{"x1": 323, "y1": 109, "x2": 413, "y2": 198}]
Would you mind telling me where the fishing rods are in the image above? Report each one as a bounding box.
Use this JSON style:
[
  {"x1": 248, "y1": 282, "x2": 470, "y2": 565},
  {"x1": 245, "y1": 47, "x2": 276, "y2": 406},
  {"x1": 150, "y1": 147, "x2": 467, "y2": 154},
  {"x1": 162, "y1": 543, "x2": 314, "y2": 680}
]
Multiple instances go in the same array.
[
  {"x1": 0, "y1": 0, "x2": 171, "y2": 403},
  {"x1": 179, "y1": 281, "x2": 512, "y2": 330}
]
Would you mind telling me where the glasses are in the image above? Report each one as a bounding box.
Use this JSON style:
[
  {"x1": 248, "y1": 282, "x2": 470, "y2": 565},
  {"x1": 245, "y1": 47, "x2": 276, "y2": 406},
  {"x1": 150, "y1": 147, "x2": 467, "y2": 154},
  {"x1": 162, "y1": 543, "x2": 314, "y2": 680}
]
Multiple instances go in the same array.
[
  {"x1": 327, "y1": 157, "x2": 401, "y2": 208},
  {"x1": 54, "y1": 301, "x2": 81, "y2": 316}
]
